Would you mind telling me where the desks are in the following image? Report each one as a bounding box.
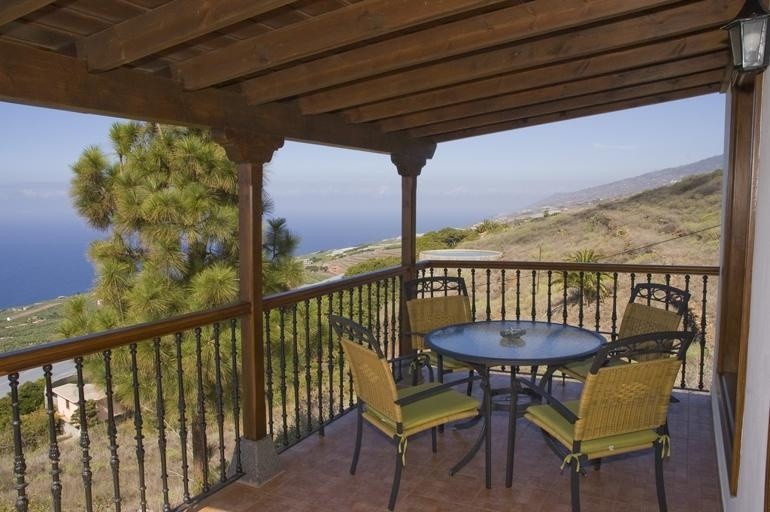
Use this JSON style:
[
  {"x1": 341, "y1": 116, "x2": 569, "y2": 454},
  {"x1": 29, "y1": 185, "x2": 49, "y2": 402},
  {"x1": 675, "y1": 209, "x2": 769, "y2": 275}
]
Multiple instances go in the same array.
[{"x1": 422, "y1": 320, "x2": 608, "y2": 477}]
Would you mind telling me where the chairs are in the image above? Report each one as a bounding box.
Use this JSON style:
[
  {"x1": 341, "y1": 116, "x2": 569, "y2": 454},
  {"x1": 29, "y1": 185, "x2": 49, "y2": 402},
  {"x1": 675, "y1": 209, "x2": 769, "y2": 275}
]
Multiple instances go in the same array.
[
  {"x1": 520, "y1": 282, "x2": 695, "y2": 511},
  {"x1": 327, "y1": 276, "x2": 491, "y2": 511},
  {"x1": 547, "y1": 282, "x2": 691, "y2": 408}
]
